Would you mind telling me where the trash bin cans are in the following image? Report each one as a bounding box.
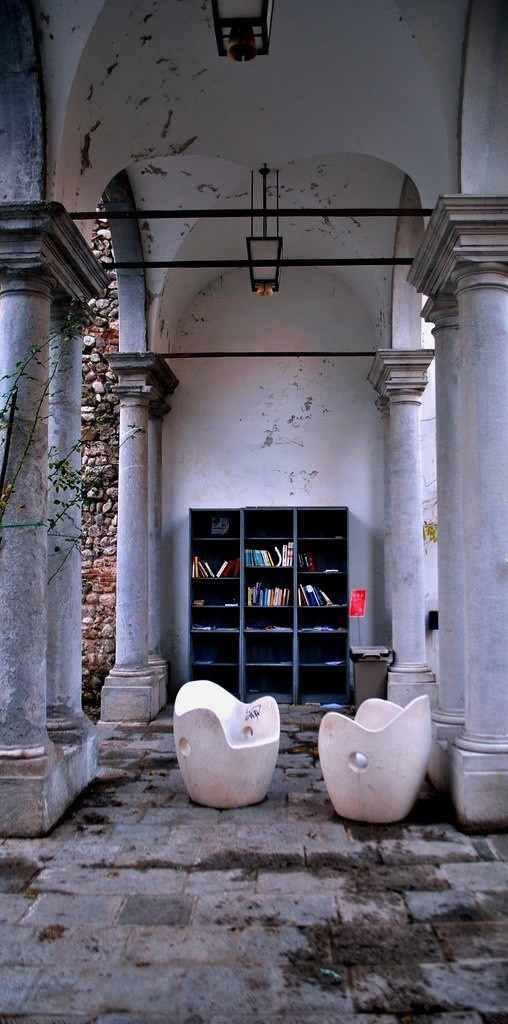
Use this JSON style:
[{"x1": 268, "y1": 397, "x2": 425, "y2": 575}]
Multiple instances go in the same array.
[{"x1": 350, "y1": 646, "x2": 389, "y2": 714}]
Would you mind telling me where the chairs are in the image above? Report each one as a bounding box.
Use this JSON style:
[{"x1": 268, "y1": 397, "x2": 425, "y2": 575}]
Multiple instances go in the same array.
[
  {"x1": 173, "y1": 679, "x2": 279, "y2": 810},
  {"x1": 317, "y1": 696, "x2": 437, "y2": 826}
]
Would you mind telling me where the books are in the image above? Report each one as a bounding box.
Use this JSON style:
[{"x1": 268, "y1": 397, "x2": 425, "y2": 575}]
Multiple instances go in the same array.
[
  {"x1": 245, "y1": 542, "x2": 294, "y2": 567},
  {"x1": 194, "y1": 659, "x2": 213, "y2": 664},
  {"x1": 225, "y1": 603, "x2": 238, "y2": 606},
  {"x1": 192, "y1": 555, "x2": 240, "y2": 579},
  {"x1": 301, "y1": 625, "x2": 336, "y2": 632},
  {"x1": 297, "y1": 584, "x2": 333, "y2": 606},
  {"x1": 266, "y1": 624, "x2": 292, "y2": 631},
  {"x1": 247, "y1": 581, "x2": 290, "y2": 606},
  {"x1": 298, "y1": 552, "x2": 315, "y2": 572},
  {"x1": 193, "y1": 624, "x2": 237, "y2": 631}
]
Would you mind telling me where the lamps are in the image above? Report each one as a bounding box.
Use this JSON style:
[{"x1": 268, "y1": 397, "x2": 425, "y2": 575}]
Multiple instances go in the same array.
[
  {"x1": 247, "y1": 167, "x2": 286, "y2": 298},
  {"x1": 211, "y1": 0, "x2": 274, "y2": 61}
]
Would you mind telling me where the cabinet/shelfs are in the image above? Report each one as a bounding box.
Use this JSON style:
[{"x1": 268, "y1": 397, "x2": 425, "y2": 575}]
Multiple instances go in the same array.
[{"x1": 186, "y1": 507, "x2": 353, "y2": 706}]
